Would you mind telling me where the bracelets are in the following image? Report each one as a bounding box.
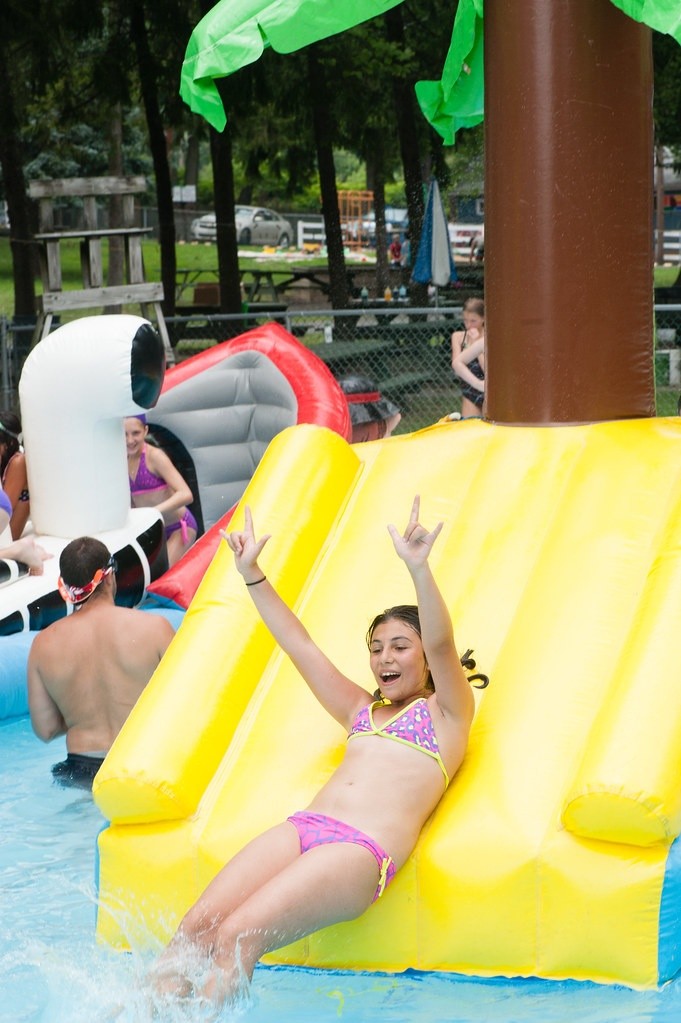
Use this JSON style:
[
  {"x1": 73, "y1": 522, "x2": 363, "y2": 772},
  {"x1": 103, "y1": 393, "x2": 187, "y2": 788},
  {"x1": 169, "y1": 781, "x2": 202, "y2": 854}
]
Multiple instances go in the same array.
[{"x1": 246, "y1": 576, "x2": 266, "y2": 585}]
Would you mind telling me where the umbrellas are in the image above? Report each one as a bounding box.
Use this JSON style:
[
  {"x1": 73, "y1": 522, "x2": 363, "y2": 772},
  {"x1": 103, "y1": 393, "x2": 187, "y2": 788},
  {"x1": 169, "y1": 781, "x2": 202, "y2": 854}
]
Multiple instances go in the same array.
[{"x1": 408, "y1": 179, "x2": 456, "y2": 347}]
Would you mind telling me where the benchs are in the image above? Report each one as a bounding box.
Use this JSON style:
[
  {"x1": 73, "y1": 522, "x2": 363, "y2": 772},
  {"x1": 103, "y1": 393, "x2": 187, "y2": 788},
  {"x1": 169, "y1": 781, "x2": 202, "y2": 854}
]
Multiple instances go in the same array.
[{"x1": 375, "y1": 371, "x2": 430, "y2": 395}]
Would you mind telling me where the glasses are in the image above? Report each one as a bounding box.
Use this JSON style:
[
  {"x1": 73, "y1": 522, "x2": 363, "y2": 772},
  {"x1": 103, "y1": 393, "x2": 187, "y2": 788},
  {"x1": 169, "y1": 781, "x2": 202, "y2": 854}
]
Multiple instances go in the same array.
[{"x1": 107, "y1": 557, "x2": 119, "y2": 572}]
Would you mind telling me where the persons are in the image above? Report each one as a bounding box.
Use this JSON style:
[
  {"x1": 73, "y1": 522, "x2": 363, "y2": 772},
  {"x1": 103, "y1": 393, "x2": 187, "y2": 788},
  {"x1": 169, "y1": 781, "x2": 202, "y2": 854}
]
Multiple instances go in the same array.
[
  {"x1": 151, "y1": 496, "x2": 476, "y2": 997},
  {"x1": 26, "y1": 536, "x2": 176, "y2": 771},
  {"x1": 124, "y1": 413, "x2": 198, "y2": 569},
  {"x1": 470, "y1": 231, "x2": 484, "y2": 264},
  {"x1": 0, "y1": 413, "x2": 54, "y2": 576},
  {"x1": 451, "y1": 335, "x2": 484, "y2": 393},
  {"x1": 389, "y1": 234, "x2": 401, "y2": 266},
  {"x1": 398, "y1": 232, "x2": 411, "y2": 266},
  {"x1": 451, "y1": 297, "x2": 486, "y2": 418}
]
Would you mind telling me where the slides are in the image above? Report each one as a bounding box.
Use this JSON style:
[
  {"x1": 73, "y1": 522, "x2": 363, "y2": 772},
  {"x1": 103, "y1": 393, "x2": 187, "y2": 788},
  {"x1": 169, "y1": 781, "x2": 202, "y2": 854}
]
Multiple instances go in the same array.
[{"x1": 86, "y1": 415, "x2": 681, "y2": 996}]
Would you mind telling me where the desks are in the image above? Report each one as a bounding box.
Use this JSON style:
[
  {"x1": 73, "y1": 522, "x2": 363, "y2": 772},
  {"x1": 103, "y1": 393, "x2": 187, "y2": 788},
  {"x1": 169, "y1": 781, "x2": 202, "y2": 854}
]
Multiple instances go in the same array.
[
  {"x1": 152, "y1": 267, "x2": 363, "y2": 303},
  {"x1": 307, "y1": 339, "x2": 411, "y2": 416},
  {"x1": 358, "y1": 318, "x2": 464, "y2": 376}
]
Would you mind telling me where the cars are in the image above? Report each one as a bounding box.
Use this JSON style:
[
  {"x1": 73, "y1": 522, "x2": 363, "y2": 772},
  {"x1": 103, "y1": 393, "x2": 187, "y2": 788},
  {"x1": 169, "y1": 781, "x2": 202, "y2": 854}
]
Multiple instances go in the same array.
[{"x1": 191, "y1": 205, "x2": 294, "y2": 248}]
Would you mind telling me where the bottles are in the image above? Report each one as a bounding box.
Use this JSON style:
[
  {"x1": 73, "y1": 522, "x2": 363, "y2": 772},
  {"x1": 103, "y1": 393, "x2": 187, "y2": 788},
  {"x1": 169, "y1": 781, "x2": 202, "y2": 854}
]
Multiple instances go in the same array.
[
  {"x1": 360, "y1": 285, "x2": 369, "y2": 304},
  {"x1": 383, "y1": 284, "x2": 407, "y2": 303}
]
